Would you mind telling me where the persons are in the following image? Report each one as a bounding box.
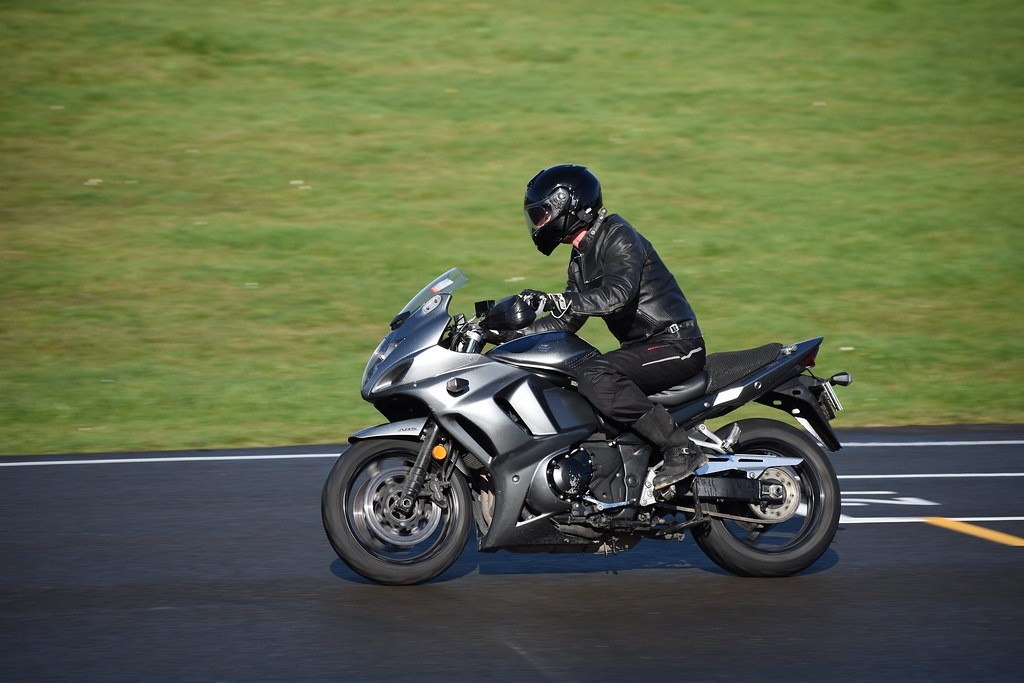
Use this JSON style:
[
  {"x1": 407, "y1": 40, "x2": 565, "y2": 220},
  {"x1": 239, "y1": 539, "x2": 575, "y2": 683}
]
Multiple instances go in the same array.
[{"x1": 482, "y1": 165, "x2": 709, "y2": 490}]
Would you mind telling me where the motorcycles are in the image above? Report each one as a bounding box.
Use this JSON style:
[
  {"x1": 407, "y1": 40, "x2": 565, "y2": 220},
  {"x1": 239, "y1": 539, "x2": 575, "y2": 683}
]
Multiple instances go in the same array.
[{"x1": 322, "y1": 266, "x2": 852, "y2": 585}]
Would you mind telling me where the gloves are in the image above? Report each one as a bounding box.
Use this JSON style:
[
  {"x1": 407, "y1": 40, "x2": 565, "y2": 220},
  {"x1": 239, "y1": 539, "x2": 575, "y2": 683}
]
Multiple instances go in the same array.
[{"x1": 519, "y1": 288, "x2": 555, "y2": 311}]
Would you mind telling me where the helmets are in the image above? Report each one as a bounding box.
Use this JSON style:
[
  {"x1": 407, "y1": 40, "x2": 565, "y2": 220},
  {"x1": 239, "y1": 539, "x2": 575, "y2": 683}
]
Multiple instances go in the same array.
[{"x1": 524, "y1": 164, "x2": 603, "y2": 255}]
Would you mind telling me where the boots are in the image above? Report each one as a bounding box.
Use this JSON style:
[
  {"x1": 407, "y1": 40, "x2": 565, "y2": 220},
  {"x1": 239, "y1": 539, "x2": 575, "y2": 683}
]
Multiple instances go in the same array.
[{"x1": 631, "y1": 403, "x2": 709, "y2": 490}]
